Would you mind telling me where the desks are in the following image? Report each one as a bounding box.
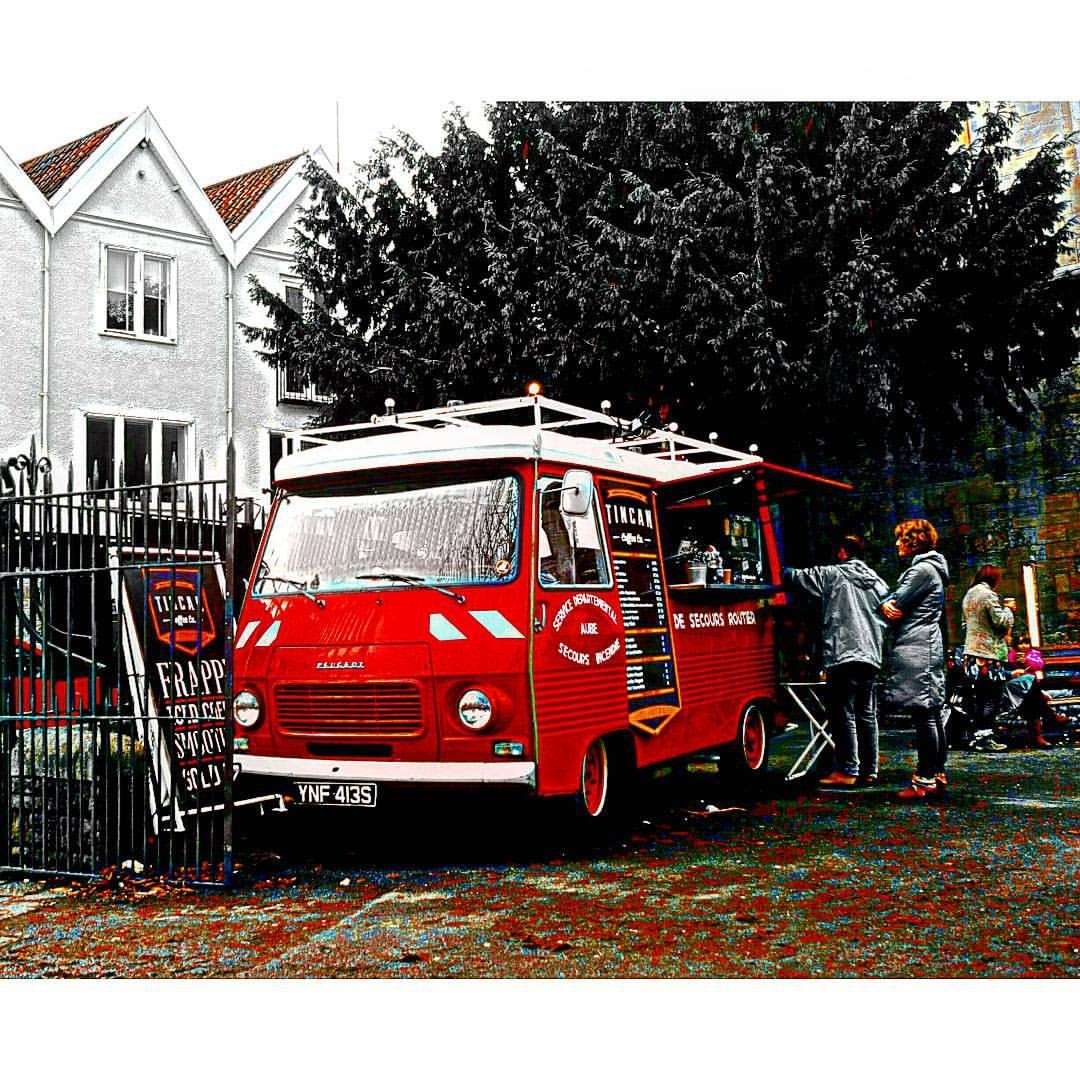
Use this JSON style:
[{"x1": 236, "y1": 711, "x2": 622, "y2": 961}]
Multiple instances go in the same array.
[{"x1": 779, "y1": 675, "x2": 848, "y2": 779}]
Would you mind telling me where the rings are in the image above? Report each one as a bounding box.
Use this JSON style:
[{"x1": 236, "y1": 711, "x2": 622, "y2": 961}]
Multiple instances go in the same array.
[{"x1": 886, "y1": 615, "x2": 891, "y2": 619}]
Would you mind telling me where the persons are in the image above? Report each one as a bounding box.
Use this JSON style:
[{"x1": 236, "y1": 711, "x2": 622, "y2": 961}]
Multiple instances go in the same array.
[
  {"x1": 877, "y1": 517, "x2": 952, "y2": 797},
  {"x1": 788, "y1": 532, "x2": 886, "y2": 786},
  {"x1": 959, "y1": 566, "x2": 1018, "y2": 747}
]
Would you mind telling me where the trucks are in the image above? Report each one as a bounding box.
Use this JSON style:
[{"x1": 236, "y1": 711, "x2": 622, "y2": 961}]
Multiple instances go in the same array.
[{"x1": 232, "y1": 394, "x2": 855, "y2": 836}]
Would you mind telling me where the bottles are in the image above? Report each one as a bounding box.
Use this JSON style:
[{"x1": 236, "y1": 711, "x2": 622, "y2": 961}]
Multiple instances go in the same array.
[{"x1": 723, "y1": 515, "x2": 760, "y2": 583}]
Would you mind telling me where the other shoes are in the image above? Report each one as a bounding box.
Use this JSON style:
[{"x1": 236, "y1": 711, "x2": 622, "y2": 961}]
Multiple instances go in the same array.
[
  {"x1": 1031, "y1": 735, "x2": 1052, "y2": 748},
  {"x1": 1044, "y1": 712, "x2": 1073, "y2": 727},
  {"x1": 896, "y1": 772, "x2": 947, "y2": 798},
  {"x1": 855, "y1": 774, "x2": 881, "y2": 785},
  {"x1": 819, "y1": 772, "x2": 856, "y2": 785},
  {"x1": 975, "y1": 739, "x2": 1008, "y2": 751}
]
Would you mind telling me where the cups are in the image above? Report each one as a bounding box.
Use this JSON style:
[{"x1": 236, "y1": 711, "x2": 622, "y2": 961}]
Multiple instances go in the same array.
[
  {"x1": 689, "y1": 566, "x2": 706, "y2": 586},
  {"x1": 1004, "y1": 598, "x2": 1015, "y2": 604}
]
